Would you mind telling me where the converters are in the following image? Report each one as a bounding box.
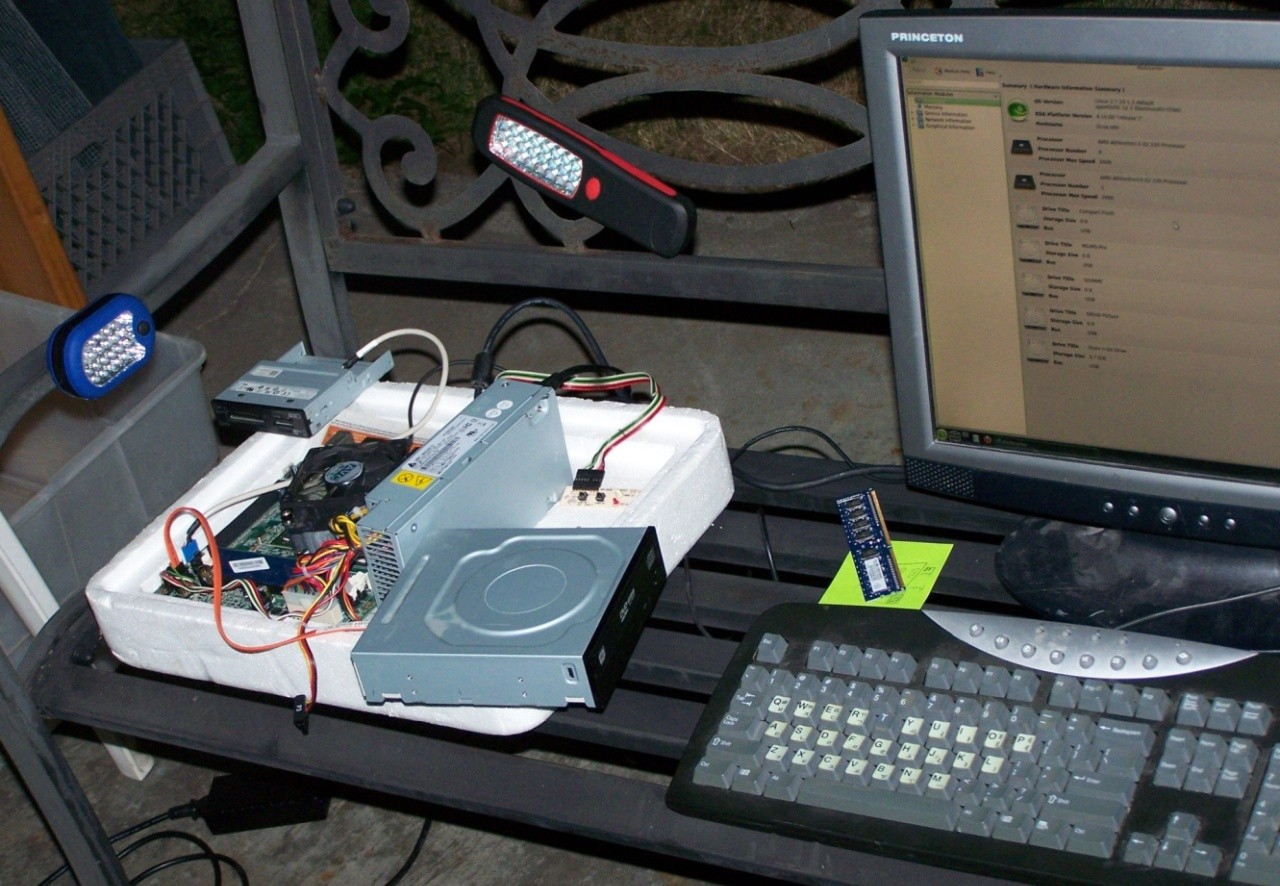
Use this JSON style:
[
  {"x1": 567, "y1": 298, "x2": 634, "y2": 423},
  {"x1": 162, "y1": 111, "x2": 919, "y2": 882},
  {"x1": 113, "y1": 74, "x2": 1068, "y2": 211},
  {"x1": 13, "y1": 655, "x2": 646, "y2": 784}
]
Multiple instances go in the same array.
[{"x1": 208, "y1": 772, "x2": 330, "y2": 836}]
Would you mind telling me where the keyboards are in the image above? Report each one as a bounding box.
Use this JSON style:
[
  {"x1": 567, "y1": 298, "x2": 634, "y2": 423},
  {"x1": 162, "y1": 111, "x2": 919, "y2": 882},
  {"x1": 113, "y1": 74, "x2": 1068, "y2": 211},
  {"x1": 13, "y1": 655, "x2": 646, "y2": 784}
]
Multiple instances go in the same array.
[{"x1": 663, "y1": 601, "x2": 1280, "y2": 886}]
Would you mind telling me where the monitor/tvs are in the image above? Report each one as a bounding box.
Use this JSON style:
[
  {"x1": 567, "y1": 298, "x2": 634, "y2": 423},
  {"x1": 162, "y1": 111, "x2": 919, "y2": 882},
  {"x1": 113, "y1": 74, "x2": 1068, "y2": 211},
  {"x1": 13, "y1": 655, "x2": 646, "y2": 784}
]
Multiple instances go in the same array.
[{"x1": 858, "y1": 8, "x2": 1280, "y2": 645}]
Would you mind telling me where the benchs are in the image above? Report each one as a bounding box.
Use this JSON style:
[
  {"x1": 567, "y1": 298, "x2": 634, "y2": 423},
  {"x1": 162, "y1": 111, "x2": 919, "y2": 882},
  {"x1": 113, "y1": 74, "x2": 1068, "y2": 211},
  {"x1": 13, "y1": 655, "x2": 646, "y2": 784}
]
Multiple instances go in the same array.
[{"x1": 0, "y1": 1, "x2": 1278, "y2": 886}]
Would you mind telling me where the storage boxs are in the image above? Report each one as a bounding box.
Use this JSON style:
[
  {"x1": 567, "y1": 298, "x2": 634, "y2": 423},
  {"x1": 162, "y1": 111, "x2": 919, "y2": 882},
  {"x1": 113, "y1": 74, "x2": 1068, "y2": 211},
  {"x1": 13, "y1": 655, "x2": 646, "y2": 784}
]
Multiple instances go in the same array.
[{"x1": 0, "y1": 290, "x2": 217, "y2": 729}]
